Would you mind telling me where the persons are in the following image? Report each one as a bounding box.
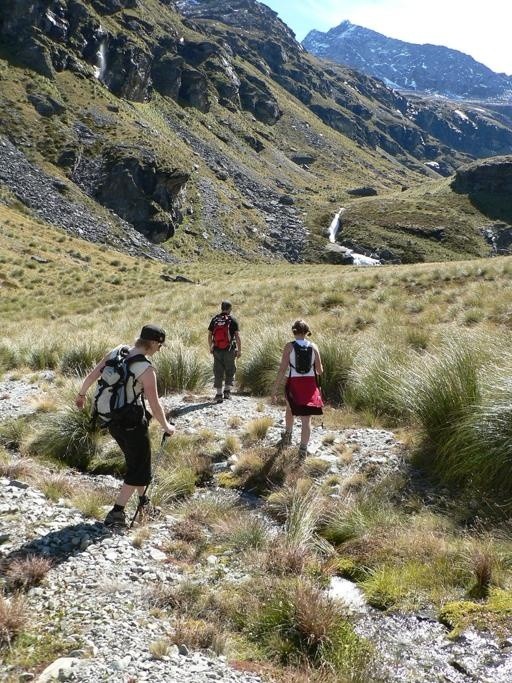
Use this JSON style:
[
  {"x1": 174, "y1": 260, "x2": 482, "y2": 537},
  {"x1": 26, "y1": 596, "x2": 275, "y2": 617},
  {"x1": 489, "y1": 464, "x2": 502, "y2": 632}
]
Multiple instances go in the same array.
[
  {"x1": 208, "y1": 296, "x2": 242, "y2": 404},
  {"x1": 73, "y1": 324, "x2": 178, "y2": 531},
  {"x1": 270, "y1": 317, "x2": 327, "y2": 460}
]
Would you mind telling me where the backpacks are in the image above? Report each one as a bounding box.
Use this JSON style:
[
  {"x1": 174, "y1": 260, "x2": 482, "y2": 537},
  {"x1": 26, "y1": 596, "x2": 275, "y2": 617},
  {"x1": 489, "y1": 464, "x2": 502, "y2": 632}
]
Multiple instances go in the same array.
[
  {"x1": 289, "y1": 340, "x2": 314, "y2": 375},
  {"x1": 211, "y1": 314, "x2": 233, "y2": 350},
  {"x1": 89, "y1": 345, "x2": 150, "y2": 434}
]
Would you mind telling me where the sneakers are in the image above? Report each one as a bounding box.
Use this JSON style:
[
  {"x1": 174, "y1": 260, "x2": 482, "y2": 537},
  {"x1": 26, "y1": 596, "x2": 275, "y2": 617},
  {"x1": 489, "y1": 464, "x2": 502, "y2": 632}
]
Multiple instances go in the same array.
[
  {"x1": 104, "y1": 510, "x2": 129, "y2": 529},
  {"x1": 281, "y1": 430, "x2": 293, "y2": 443},
  {"x1": 139, "y1": 502, "x2": 162, "y2": 522},
  {"x1": 214, "y1": 390, "x2": 232, "y2": 401}
]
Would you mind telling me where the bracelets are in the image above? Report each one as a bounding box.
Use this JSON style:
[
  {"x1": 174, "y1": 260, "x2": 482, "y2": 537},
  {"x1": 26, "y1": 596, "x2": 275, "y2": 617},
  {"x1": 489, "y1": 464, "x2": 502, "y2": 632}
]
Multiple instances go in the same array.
[{"x1": 78, "y1": 392, "x2": 87, "y2": 397}]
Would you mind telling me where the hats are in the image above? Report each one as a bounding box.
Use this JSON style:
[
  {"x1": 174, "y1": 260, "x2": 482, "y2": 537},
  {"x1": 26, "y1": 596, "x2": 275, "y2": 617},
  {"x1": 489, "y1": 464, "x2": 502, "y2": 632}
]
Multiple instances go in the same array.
[{"x1": 140, "y1": 325, "x2": 166, "y2": 343}]
